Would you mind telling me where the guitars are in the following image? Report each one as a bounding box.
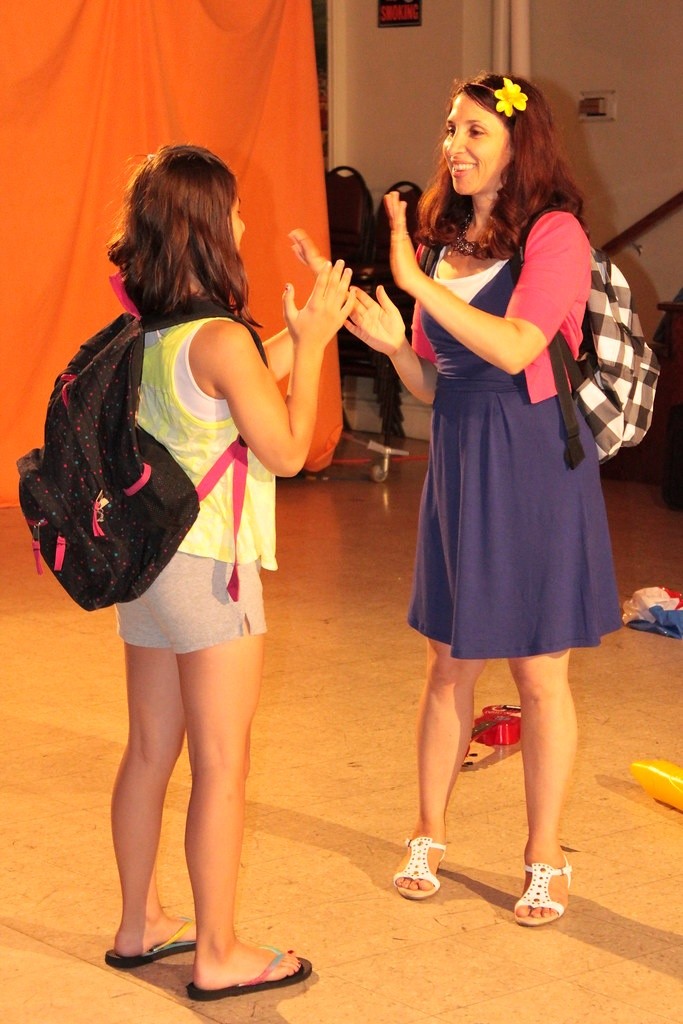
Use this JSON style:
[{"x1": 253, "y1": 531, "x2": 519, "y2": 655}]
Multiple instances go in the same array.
[{"x1": 465, "y1": 704, "x2": 521, "y2": 759}]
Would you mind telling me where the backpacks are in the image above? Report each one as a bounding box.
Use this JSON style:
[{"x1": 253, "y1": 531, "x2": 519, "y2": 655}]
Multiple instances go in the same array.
[
  {"x1": 17, "y1": 294, "x2": 268, "y2": 612},
  {"x1": 419, "y1": 203, "x2": 661, "y2": 464}
]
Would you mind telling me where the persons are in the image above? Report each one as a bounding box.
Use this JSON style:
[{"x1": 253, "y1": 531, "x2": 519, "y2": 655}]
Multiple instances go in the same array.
[
  {"x1": 104, "y1": 143, "x2": 357, "y2": 1001},
  {"x1": 342, "y1": 73, "x2": 626, "y2": 927}
]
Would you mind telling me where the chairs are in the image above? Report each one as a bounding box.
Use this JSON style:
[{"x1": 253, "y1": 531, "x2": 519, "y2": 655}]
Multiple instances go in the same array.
[
  {"x1": 325, "y1": 165, "x2": 376, "y2": 265},
  {"x1": 376, "y1": 181, "x2": 423, "y2": 262}
]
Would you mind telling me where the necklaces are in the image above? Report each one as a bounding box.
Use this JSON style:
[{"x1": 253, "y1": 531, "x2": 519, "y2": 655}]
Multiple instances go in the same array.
[{"x1": 455, "y1": 209, "x2": 483, "y2": 255}]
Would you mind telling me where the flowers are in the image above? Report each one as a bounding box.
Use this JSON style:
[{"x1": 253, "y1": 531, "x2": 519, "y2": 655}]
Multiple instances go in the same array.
[{"x1": 493, "y1": 77, "x2": 528, "y2": 117}]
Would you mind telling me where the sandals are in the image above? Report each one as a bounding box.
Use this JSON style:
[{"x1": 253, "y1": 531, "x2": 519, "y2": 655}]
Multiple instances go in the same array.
[
  {"x1": 393, "y1": 836, "x2": 447, "y2": 900},
  {"x1": 514, "y1": 854, "x2": 573, "y2": 925}
]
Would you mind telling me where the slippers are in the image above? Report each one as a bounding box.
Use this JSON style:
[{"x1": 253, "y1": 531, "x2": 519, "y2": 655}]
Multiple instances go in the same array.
[
  {"x1": 186, "y1": 945, "x2": 313, "y2": 1001},
  {"x1": 105, "y1": 916, "x2": 197, "y2": 969}
]
